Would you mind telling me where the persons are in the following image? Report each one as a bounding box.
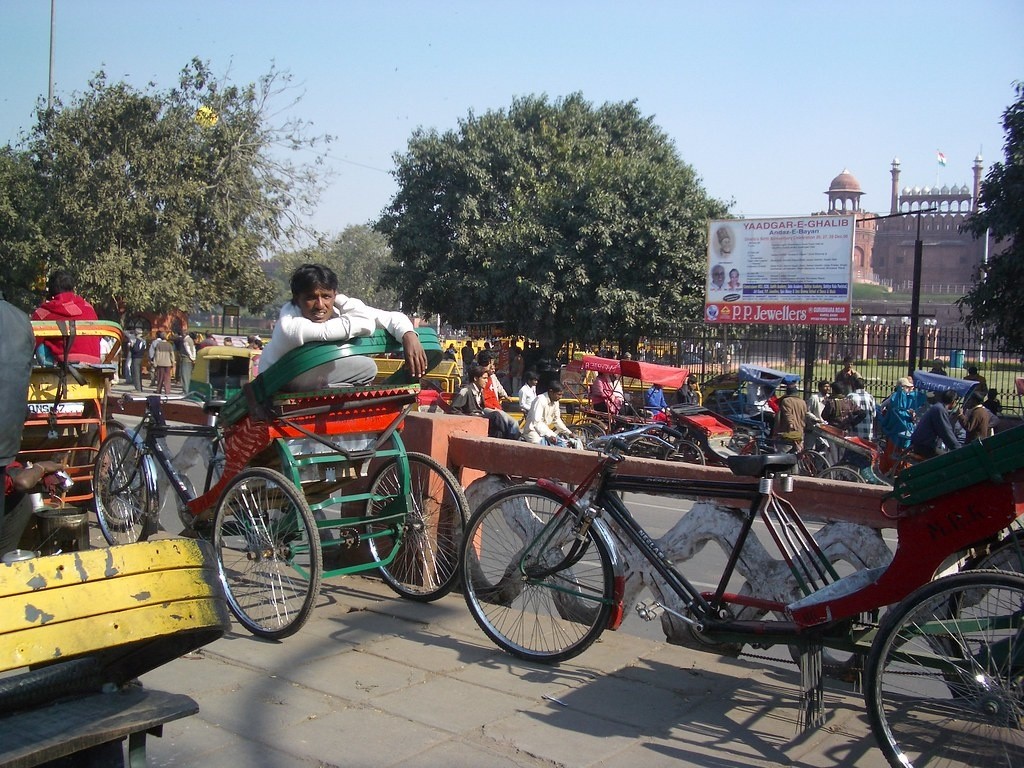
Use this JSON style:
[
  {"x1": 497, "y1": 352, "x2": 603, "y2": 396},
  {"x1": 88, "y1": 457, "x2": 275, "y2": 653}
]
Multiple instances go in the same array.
[
  {"x1": 123, "y1": 328, "x2": 146, "y2": 392},
  {"x1": 30, "y1": 271, "x2": 102, "y2": 365},
  {"x1": 518, "y1": 371, "x2": 540, "y2": 419},
  {"x1": 524, "y1": 381, "x2": 579, "y2": 448},
  {"x1": 710, "y1": 265, "x2": 727, "y2": 290},
  {"x1": 644, "y1": 383, "x2": 671, "y2": 443},
  {"x1": 591, "y1": 370, "x2": 625, "y2": 432},
  {"x1": 0, "y1": 262, "x2": 35, "y2": 535},
  {"x1": 224, "y1": 337, "x2": 233, "y2": 346},
  {"x1": 449, "y1": 358, "x2": 523, "y2": 441},
  {"x1": 245, "y1": 335, "x2": 264, "y2": 380},
  {"x1": 462, "y1": 341, "x2": 568, "y2": 397},
  {"x1": 0, "y1": 407, "x2": 66, "y2": 563},
  {"x1": 746, "y1": 356, "x2": 1001, "y2": 485},
  {"x1": 259, "y1": 264, "x2": 428, "y2": 392},
  {"x1": 675, "y1": 374, "x2": 698, "y2": 453},
  {"x1": 100, "y1": 334, "x2": 122, "y2": 384},
  {"x1": 572, "y1": 344, "x2": 731, "y2": 366},
  {"x1": 148, "y1": 330, "x2": 217, "y2": 396},
  {"x1": 443, "y1": 343, "x2": 461, "y2": 392},
  {"x1": 728, "y1": 268, "x2": 741, "y2": 288}
]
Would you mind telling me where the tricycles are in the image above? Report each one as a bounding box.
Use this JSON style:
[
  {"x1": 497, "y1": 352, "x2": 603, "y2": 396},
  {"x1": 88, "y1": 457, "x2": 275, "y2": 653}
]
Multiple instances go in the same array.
[
  {"x1": 0, "y1": 534, "x2": 233, "y2": 767},
  {"x1": 96, "y1": 325, "x2": 473, "y2": 641},
  {"x1": 372, "y1": 350, "x2": 1024, "y2": 541},
  {"x1": 12, "y1": 289, "x2": 149, "y2": 532},
  {"x1": 459, "y1": 420, "x2": 1023, "y2": 768}
]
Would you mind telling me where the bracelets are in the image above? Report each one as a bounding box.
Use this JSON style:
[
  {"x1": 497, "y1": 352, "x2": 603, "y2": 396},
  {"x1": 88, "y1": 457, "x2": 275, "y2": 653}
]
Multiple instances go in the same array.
[{"x1": 35, "y1": 464, "x2": 45, "y2": 471}]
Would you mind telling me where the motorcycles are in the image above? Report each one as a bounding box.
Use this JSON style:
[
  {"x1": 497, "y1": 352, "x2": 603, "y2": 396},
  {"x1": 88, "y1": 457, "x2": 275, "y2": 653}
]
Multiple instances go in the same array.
[{"x1": 186, "y1": 346, "x2": 264, "y2": 401}]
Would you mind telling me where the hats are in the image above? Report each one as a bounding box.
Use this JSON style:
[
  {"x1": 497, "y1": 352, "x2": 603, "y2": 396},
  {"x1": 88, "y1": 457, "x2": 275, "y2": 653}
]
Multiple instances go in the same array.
[
  {"x1": 830, "y1": 382, "x2": 843, "y2": 393},
  {"x1": 897, "y1": 378, "x2": 913, "y2": 387}
]
